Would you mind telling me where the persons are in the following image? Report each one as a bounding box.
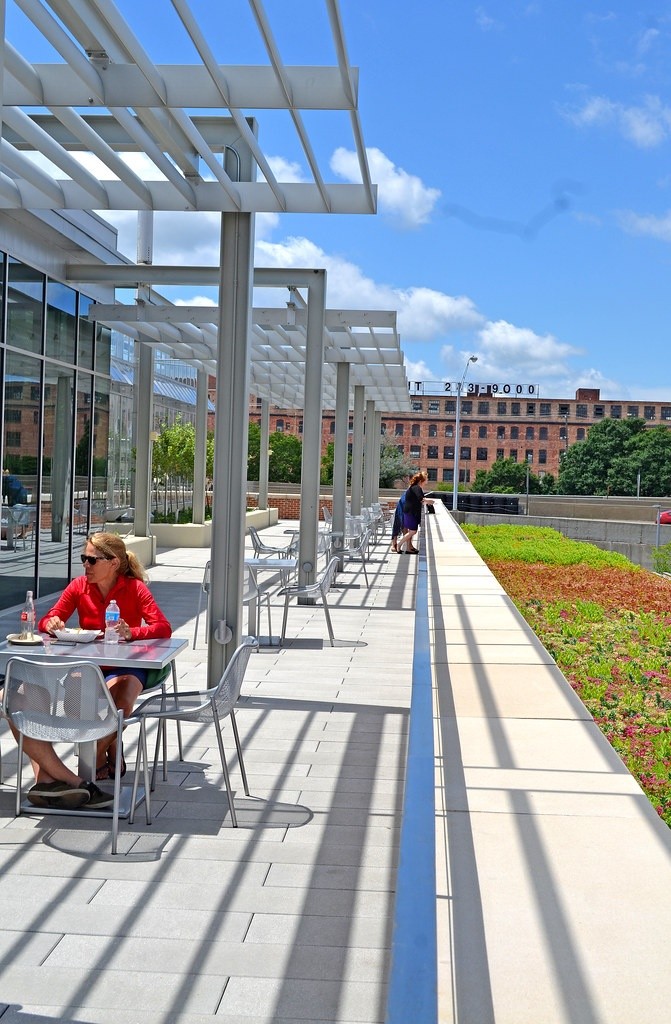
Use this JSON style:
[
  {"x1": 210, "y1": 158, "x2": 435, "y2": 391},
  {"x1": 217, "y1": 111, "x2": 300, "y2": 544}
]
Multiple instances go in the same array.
[
  {"x1": 0, "y1": 673, "x2": 115, "y2": 808},
  {"x1": 2, "y1": 469, "x2": 27, "y2": 539},
  {"x1": 391, "y1": 471, "x2": 435, "y2": 555},
  {"x1": 38, "y1": 530, "x2": 172, "y2": 781}
]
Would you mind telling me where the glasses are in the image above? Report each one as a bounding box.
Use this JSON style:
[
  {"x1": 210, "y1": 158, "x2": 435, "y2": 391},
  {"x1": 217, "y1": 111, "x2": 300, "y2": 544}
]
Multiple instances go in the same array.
[{"x1": 81, "y1": 554, "x2": 113, "y2": 566}]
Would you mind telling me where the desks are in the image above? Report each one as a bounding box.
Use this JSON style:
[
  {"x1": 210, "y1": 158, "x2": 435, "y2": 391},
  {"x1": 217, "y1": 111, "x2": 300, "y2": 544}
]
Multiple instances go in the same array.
[
  {"x1": 2, "y1": 505, "x2": 37, "y2": 551},
  {"x1": 242, "y1": 558, "x2": 299, "y2": 647},
  {"x1": 281, "y1": 528, "x2": 344, "y2": 572},
  {"x1": 0, "y1": 630, "x2": 189, "y2": 818}
]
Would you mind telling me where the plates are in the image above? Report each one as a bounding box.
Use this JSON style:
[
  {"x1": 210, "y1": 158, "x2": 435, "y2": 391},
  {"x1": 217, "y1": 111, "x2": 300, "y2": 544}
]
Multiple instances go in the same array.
[
  {"x1": 6, "y1": 633, "x2": 43, "y2": 645},
  {"x1": 54, "y1": 629, "x2": 101, "y2": 642}
]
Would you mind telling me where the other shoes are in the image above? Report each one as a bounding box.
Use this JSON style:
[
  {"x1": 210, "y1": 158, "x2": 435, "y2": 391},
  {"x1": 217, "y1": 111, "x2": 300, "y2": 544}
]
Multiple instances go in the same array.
[
  {"x1": 394, "y1": 544, "x2": 404, "y2": 552},
  {"x1": 395, "y1": 545, "x2": 402, "y2": 554},
  {"x1": 411, "y1": 548, "x2": 419, "y2": 552},
  {"x1": 405, "y1": 551, "x2": 418, "y2": 554},
  {"x1": 17, "y1": 535, "x2": 27, "y2": 539}
]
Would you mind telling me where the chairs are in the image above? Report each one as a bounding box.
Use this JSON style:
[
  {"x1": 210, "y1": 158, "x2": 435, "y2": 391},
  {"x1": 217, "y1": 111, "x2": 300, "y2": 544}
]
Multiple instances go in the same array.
[
  {"x1": 192, "y1": 501, "x2": 395, "y2": 650},
  {"x1": 0, "y1": 656, "x2": 153, "y2": 854},
  {"x1": 122, "y1": 636, "x2": 260, "y2": 828}
]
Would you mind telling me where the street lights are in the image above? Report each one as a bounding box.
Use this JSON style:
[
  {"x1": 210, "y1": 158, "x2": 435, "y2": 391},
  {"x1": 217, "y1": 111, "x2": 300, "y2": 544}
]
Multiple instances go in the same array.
[
  {"x1": 651, "y1": 505, "x2": 661, "y2": 558},
  {"x1": 452, "y1": 354, "x2": 478, "y2": 512}
]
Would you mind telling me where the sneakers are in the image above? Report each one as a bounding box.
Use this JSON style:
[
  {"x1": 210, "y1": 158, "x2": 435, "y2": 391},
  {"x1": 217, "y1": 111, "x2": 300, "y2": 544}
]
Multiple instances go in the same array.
[
  {"x1": 28, "y1": 781, "x2": 90, "y2": 809},
  {"x1": 77, "y1": 781, "x2": 114, "y2": 809}
]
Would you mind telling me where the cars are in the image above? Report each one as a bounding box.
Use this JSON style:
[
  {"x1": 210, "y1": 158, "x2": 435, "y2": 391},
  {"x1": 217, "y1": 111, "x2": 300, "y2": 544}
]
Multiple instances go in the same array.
[{"x1": 655, "y1": 510, "x2": 671, "y2": 524}]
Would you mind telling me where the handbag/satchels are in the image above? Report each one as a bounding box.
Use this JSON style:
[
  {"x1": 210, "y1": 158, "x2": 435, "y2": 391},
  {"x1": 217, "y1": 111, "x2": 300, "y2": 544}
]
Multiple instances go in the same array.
[{"x1": 426, "y1": 502, "x2": 435, "y2": 514}]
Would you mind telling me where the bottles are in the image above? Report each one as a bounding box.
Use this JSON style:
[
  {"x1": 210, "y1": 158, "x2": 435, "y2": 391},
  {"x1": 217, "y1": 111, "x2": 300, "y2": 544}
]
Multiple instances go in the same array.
[
  {"x1": 21, "y1": 590, "x2": 35, "y2": 641},
  {"x1": 105, "y1": 600, "x2": 120, "y2": 644}
]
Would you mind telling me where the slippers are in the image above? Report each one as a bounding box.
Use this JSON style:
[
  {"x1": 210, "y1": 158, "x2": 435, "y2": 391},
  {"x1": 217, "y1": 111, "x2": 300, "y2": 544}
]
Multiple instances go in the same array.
[
  {"x1": 95, "y1": 765, "x2": 108, "y2": 782},
  {"x1": 107, "y1": 740, "x2": 126, "y2": 779}
]
